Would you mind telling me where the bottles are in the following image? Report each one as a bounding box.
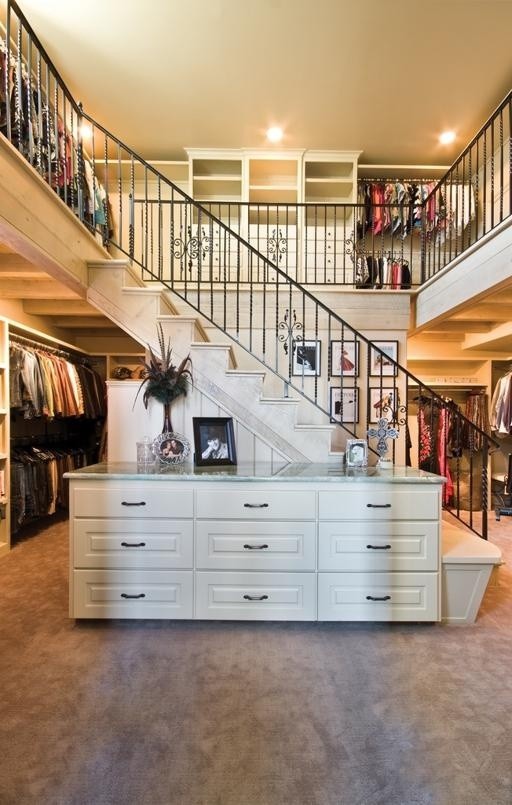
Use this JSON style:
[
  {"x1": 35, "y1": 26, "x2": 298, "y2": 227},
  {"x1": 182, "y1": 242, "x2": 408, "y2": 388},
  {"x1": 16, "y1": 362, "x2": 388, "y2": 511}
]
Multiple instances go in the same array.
[{"x1": 137, "y1": 440, "x2": 154, "y2": 464}]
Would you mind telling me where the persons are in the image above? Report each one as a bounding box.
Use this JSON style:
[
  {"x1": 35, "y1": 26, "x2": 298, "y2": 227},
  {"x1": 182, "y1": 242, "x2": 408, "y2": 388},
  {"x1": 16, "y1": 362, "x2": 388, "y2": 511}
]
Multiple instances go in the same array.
[
  {"x1": 170, "y1": 440, "x2": 180, "y2": 454},
  {"x1": 162, "y1": 442, "x2": 173, "y2": 455},
  {"x1": 201, "y1": 431, "x2": 228, "y2": 459}
]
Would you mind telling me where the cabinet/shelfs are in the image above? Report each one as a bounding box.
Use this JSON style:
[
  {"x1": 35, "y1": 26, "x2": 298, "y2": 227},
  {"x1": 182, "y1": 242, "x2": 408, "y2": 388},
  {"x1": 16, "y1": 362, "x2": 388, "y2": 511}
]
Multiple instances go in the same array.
[
  {"x1": 0, "y1": 318, "x2": 148, "y2": 557},
  {"x1": 185, "y1": 149, "x2": 364, "y2": 288},
  {"x1": 407, "y1": 356, "x2": 512, "y2": 513},
  {"x1": 64, "y1": 480, "x2": 446, "y2": 625}
]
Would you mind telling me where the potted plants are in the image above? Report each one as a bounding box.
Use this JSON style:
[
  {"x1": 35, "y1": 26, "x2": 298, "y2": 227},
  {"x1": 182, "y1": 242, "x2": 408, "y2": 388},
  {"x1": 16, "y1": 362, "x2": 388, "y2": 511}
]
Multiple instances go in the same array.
[{"x1": 132, "y1": 323, "x2": 194, "y2": 434}]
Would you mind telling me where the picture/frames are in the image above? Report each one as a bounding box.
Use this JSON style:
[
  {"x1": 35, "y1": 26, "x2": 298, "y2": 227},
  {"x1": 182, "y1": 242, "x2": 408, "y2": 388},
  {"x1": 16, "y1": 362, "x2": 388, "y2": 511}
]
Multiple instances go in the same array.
[
  {"x1": 192, "y1": 416, "x2": 237, "y2": 466},
  {"x1": 289, "y1": 339, "x2": 399, "y2": 425}
]
